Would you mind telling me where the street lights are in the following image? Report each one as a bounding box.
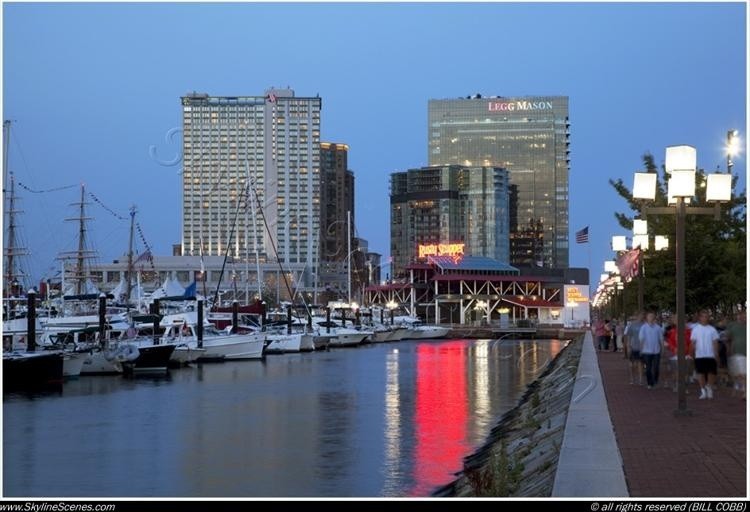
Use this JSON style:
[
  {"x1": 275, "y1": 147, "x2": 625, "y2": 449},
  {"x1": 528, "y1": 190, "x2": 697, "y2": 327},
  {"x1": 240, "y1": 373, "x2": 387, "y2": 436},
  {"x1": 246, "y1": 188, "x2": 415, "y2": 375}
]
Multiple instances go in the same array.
[
  {"x1": 473, "y1": 300, "x2": 488, "y2": 328},
  {"x1": 567, "y1": 299, "x2": 581, "y2": 331},
  {"x1": 591, "y1": 144, "x2": 732, "y2": 418}
]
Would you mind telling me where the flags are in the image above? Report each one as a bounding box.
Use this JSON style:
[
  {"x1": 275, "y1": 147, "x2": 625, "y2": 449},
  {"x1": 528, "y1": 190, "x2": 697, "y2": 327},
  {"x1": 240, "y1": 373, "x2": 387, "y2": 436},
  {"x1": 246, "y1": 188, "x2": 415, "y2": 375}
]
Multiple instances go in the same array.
[{"x1": 575, "y1": 227, "x2": 587, "y2": 242}]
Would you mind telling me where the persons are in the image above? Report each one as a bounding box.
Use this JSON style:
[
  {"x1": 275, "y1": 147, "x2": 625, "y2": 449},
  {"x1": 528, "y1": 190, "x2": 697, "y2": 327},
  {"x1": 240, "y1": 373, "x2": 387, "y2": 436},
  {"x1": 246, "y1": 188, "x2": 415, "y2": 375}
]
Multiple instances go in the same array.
[{"x1": 595, "y1": 310, "x2": 746, "y2": 400}]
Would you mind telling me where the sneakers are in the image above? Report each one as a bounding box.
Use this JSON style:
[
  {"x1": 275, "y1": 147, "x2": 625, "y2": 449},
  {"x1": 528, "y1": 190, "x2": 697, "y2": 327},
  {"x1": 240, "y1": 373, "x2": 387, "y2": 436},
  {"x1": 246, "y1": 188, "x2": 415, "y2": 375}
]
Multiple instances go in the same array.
[
  {"x1": 707, "y1": 391, "x2": 714, "y2": 399},
  {"x1": 628, "y1": 377, "x2": 695, "y2": 395},
  {"x1": 699, "y1": 390, "x2": 707, "y2": 400}
]
